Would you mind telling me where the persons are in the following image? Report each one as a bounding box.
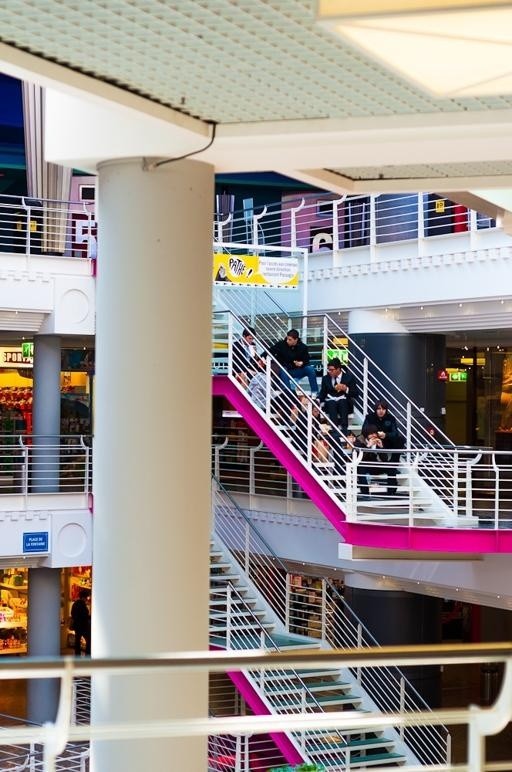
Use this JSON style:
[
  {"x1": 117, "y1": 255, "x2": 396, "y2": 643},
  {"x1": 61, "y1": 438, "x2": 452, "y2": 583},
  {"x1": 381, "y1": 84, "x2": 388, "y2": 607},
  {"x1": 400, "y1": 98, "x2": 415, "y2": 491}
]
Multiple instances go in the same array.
[
  {"x1": 71, "y1": 590, "x2": 91, "y2": 655},
  {"x1": 233, "y1": 327, "x2": 407, "y2": 501}
]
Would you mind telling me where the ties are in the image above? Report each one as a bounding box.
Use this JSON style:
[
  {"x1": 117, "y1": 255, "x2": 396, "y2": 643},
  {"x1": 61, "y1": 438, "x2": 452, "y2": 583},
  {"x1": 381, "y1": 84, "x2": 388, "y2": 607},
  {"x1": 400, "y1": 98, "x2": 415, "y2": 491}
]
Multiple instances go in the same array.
[{"x1": 334, "y1": 376, "x2": 338, "y2": 385}]
